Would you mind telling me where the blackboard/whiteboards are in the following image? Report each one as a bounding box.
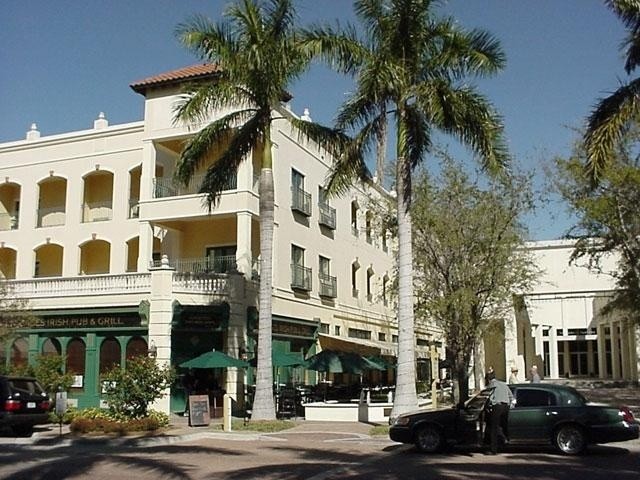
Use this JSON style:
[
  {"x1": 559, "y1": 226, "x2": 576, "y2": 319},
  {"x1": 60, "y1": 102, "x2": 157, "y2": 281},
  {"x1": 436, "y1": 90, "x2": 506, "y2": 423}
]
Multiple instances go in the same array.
[{"x1": 189, "y1": 395, "x2": 210, "y2": 426}]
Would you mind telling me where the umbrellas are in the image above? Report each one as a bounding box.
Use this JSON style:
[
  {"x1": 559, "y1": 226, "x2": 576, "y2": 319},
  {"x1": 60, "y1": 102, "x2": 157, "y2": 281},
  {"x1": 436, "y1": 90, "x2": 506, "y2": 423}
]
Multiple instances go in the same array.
[
  {"x1": 179, "y1": 350, "x2": 252, "y2": 418},
  {"x1": 293, "y1": 348, "x2": 399, "y2": 401},
  {"x1": 242, "y1": 349, "x2": 306, "y2": 412}
]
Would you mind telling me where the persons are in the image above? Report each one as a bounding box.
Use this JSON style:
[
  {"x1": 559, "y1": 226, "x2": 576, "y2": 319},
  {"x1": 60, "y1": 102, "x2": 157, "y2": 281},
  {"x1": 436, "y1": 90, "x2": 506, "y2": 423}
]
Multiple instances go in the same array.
[
  {"x1": 485, "y1": 364, "x2": 497, "y2": 386},
  {"x1": 509, "y1": 366, "x2": 522, "y2": 385},
  {"x1": 485, "y1": 372, "x2": 516, "y2": 455},
  {"x1": 528, "y1": 364, "x2": 544, "y2": 384}
]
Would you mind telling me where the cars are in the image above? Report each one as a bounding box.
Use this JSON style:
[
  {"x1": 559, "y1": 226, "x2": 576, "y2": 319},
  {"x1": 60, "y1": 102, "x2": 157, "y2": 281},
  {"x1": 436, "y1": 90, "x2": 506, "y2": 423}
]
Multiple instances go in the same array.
[{"x1": 388, "y1": 382, "x2": 640, "y2": 457}]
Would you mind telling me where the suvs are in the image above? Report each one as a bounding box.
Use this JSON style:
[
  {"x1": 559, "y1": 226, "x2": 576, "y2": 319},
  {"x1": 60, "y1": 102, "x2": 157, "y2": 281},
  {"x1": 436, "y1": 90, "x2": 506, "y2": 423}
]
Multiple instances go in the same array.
[{"x1": 0, "y1": 375, "x2": 51, "y2": 439}]
